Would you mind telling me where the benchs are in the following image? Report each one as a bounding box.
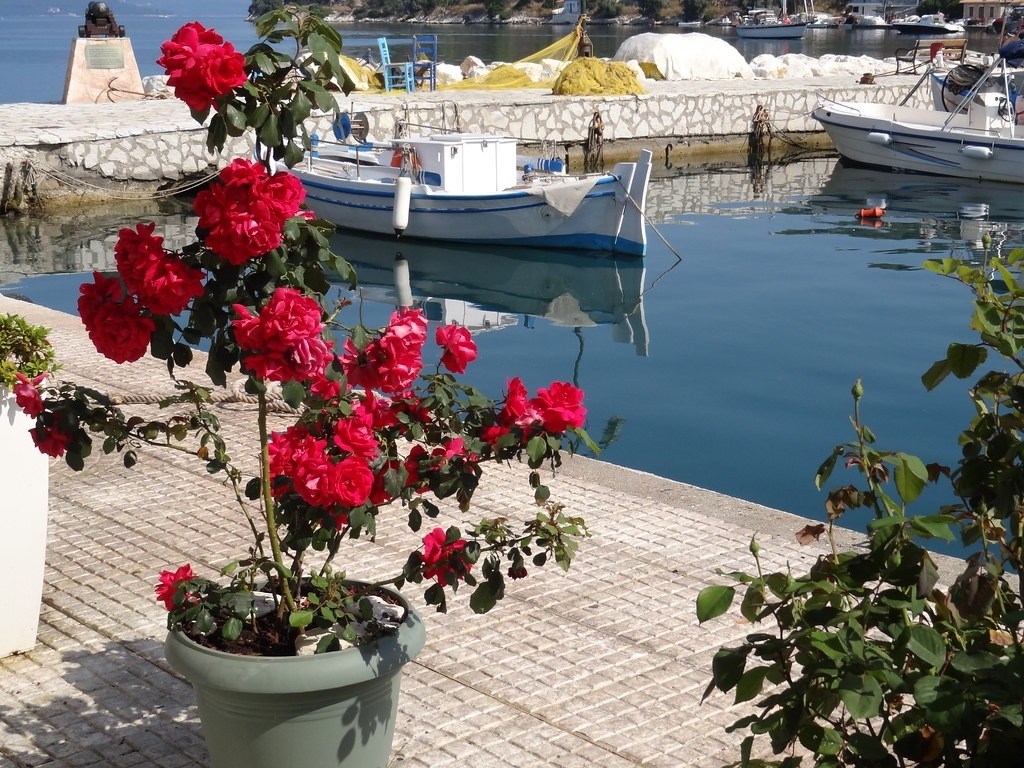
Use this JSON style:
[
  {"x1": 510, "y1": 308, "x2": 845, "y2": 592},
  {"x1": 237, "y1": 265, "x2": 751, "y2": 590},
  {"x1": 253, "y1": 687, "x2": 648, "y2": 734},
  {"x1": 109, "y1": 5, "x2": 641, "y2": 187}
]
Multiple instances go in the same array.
[{"x1": 895, "y1": 39, "x2": 969, "y2": 76}]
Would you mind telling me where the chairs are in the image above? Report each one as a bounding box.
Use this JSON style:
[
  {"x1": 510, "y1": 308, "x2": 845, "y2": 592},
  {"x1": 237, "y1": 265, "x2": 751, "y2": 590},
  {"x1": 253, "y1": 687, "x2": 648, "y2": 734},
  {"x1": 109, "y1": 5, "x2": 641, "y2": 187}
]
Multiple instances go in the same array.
[
  {"x1": 412, "y1": 35, "x2": 438, "y2": 91},
  {"x1": 377, "y1": 36, "x2": 415, "y2": 94}
]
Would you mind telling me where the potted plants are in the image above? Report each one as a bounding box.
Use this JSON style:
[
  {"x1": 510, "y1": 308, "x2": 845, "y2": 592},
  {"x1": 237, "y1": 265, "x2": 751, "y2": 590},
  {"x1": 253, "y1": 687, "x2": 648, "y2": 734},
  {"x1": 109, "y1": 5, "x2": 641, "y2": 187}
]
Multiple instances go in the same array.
[{"x1": 0, "y1": 313, "x2": 65, "y2": 659}]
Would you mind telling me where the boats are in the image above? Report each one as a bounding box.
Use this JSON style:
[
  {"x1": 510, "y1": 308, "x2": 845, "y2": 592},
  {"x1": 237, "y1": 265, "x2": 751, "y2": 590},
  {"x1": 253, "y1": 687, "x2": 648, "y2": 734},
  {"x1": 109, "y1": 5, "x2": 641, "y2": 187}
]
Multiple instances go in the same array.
[
  {"x1": 735, "y1": 15, "x2": 808, "y2": 38},
  {"x1": 320, "y1": 11, "x2": 1020, "y2": 34},
  {"x1": 925, "y1": 67, "x2": 1023, "y2": 123},
  {"x1": 247, "y1": 108, "x2": 657, "y2": 256},
  {"x1": 811, "y1": 56, "x2": 1023, "y2": 183}
]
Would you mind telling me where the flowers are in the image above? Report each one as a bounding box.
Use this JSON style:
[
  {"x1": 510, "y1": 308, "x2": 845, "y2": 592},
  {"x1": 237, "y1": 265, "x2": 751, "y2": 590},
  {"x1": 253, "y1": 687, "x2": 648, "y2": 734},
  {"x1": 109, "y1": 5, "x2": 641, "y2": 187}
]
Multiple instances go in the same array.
[{"x1": 10, "y1": 0, "x2": 625, "y2": 656}]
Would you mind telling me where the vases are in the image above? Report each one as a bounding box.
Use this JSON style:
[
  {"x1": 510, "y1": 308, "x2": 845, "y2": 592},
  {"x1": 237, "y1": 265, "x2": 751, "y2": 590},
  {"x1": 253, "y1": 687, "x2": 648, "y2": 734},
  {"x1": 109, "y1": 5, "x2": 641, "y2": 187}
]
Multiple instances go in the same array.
[{"x1": 165, "y1": 576, "x2": 426, "y2": 768}]
